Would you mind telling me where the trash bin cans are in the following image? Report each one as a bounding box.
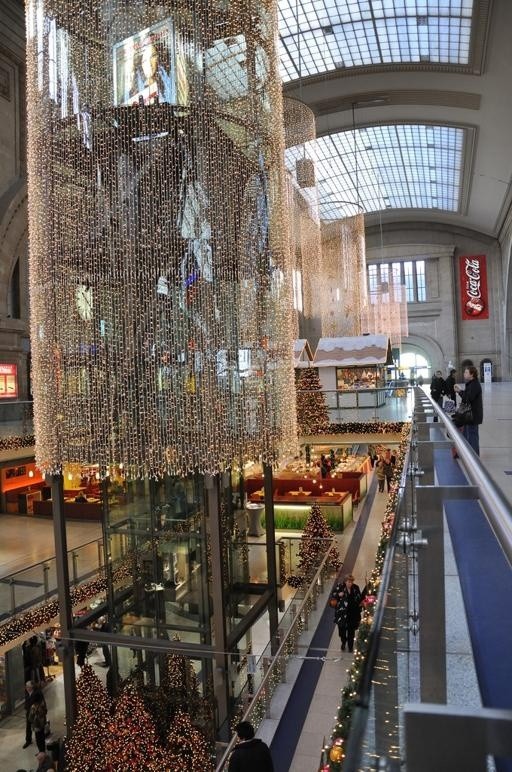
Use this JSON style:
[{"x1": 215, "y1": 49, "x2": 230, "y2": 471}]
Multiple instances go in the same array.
[{"x1": 480, "y1": 358, "x2": 492, "y2": 383}]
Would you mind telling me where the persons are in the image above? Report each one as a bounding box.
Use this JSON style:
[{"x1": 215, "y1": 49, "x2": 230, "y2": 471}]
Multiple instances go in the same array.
[
  {"x1": 429, "y1": 364, "x2": 483, "y2": 460},
  {"x1": 172, "y1": 479, "x2": 188, "y2": 529},
  {"x1": 321, "y1": 445, "x2": 397, "y2": 494},
  {"x1": 15, "y1": 615, "x2": 108, "y2": 772},
  {"x1": 331, "y1": 574, "x2": 364, "y2": 653},
  {"x1": 228, "y1": 721, "x2": 275, "y2": 771},
  {"x1": 73, "y1": 490, "x2": 88, "y2": 504},
  {"x1": 117, "y1": 25, "x2": 173, "y2": 109}
]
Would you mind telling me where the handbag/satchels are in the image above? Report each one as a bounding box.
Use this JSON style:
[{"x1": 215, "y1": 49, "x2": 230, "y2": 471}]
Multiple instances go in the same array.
[
  {"x1": 453, "y1": 403, "x2": 475, "y2": 426},
  {"x1": 328, "y1": 598, "x2": 337, "y2": 608},
  {"x1": 444, "y1": 399, "x2": 455, "y2": 414}
]
